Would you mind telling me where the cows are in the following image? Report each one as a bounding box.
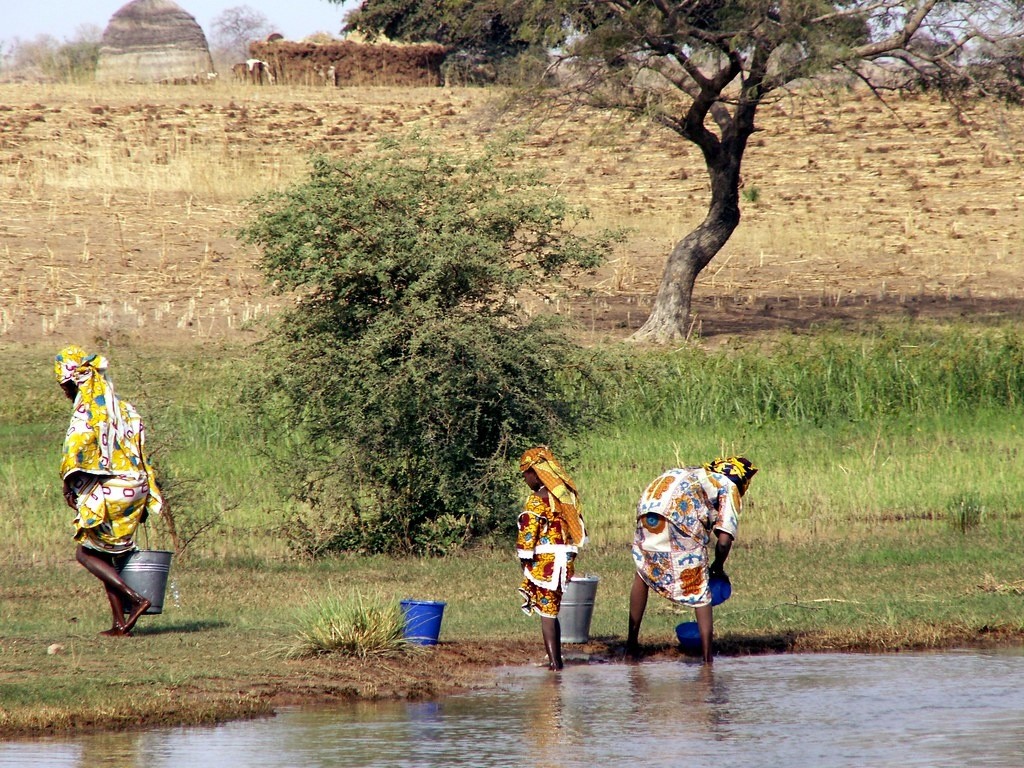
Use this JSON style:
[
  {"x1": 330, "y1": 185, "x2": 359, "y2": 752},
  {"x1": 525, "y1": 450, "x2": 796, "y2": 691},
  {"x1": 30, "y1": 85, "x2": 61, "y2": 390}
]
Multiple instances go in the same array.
[
  {"x1": 313, "y1": 63, "x2": 338, "y2": 86},
  {"x1": 154, "y1": 58, "x2": 275, "y2": 87}
]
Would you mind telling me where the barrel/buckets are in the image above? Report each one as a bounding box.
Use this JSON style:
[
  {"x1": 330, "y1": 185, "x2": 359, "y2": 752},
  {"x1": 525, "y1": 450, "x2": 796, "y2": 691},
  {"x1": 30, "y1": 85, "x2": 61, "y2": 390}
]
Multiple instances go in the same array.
[
  {"x1": 557, "y1": 575, "x2": 599, "y2": 643},
  {"x1": 708, "y1": 572, "x2": 732, "y2": 607},
  {"x1": 116, "y1": 518, "x2": 174, "y2": 614},
  {"x1": 399, "y1": 599, "x2": 447, "y2": 645},
  {"x1": 675, "y1": 622, "x2": 701, "y2": 648}
]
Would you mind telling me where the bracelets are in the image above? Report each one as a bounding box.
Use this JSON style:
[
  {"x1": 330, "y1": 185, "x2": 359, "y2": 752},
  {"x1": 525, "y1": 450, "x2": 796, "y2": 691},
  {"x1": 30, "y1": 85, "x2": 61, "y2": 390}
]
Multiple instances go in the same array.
[{"x1": 63, "y1": 487, "x2": 72, "y2": 498}]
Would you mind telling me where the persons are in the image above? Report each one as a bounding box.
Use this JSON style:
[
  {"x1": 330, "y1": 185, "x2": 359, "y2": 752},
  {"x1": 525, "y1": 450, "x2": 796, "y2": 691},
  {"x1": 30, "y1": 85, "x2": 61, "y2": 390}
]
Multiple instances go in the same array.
[
  {"x1": 626, "y1": 456, "x2": 759, "y2": 660},
  {"x1": 515, "y1": 447, "x2": 588, "y2": 672},
  {"x1": 54, "y1": 346, "x2": 164, "y2": 637}
]
